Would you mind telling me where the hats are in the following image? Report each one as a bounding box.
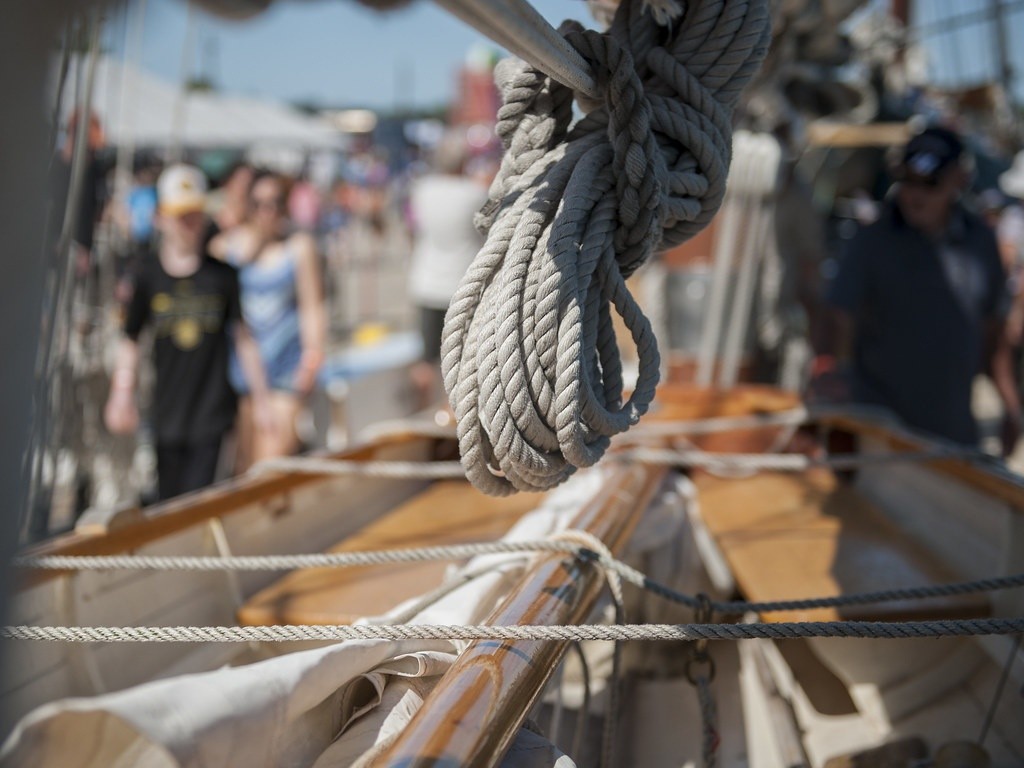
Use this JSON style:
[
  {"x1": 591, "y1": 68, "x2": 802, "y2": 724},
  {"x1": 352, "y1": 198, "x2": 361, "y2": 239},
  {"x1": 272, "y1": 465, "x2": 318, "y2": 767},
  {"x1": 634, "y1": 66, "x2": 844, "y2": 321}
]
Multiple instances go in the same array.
[
  {"x1": 157, "y1": 163, "x2": 209, "y2": 217},
  {"x1": 895, "y1": 126, "x2": 968, "y2": 190}
]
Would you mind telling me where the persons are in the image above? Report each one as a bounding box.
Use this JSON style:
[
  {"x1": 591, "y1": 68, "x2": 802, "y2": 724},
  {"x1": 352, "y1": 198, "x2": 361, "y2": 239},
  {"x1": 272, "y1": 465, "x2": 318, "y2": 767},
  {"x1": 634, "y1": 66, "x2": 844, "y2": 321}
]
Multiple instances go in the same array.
[
  {"x1": 780, "y1": 96, "x2": 1023, "y2": 462},
  {"x1": 57, "y1": 113, "x2": 501, "y2": 511}
]
